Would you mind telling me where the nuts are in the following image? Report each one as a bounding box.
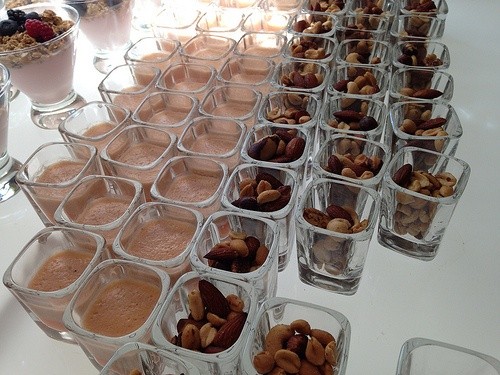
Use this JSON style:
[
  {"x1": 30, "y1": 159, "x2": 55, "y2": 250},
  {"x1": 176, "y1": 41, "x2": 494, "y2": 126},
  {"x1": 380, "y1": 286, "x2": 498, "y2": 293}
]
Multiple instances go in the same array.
[
  {"x1": 254, "y1": 319, "x2": 339, "y2": 375},
  {"x1": 167, "y1": 279, "x2": 248, "y2": 353},
  {"x1": 203, "y1": 0, "x2": 457, "y2": 276}
]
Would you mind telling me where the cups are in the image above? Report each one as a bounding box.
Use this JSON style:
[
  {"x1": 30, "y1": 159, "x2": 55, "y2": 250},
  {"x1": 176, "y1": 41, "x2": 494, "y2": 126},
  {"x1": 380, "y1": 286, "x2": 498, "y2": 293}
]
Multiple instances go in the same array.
[{"x1": 0, "y1": 1, "x2": 500, "y2": 375}]
